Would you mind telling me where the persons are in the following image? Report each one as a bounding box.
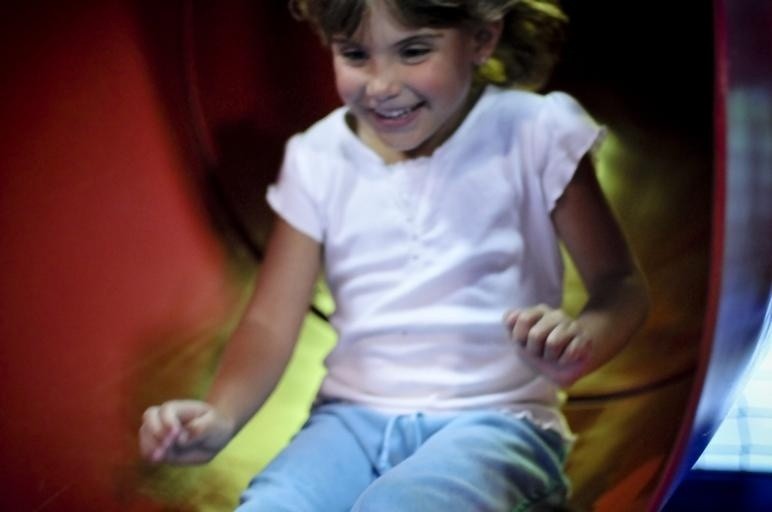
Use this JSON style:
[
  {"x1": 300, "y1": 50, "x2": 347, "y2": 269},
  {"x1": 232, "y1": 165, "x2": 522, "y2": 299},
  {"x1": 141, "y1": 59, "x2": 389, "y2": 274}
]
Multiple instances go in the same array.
[{"x1": 138, "y1": 0, "x2": 648, "y2": 511}]
